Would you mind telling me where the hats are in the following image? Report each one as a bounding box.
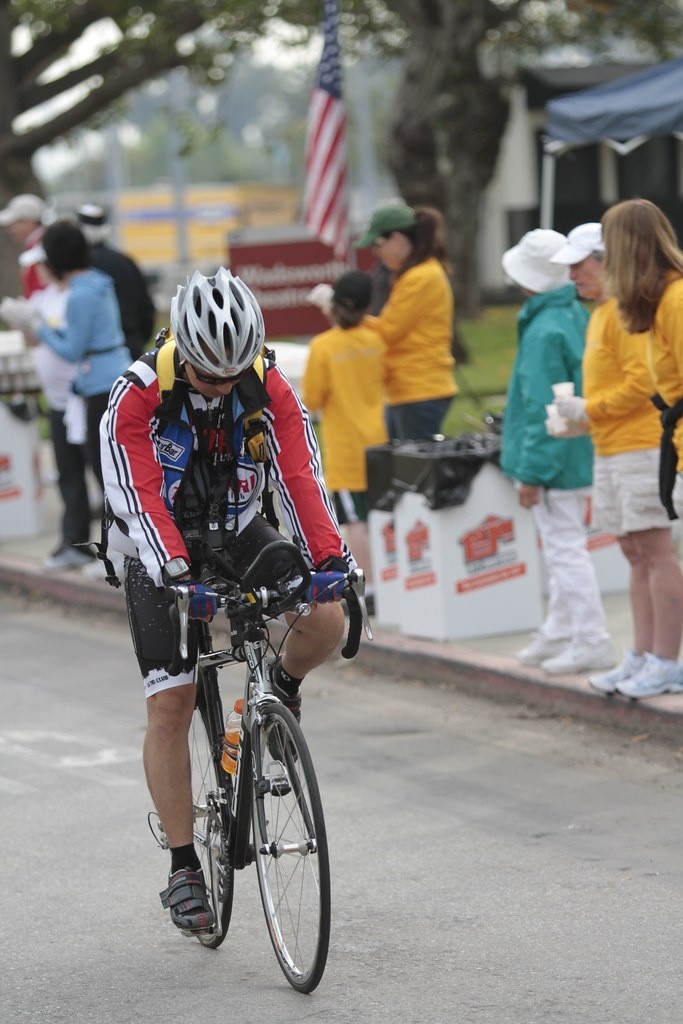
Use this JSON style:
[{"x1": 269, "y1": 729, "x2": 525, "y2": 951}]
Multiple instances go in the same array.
[
  {"x1": 501, "y1": 227, "x2": 572, "y2": 294},
  {"x1": 77, "y1": 204, "x2": 109, "y2": 242},
  {"x1": 332, "y1": 271, "x2": 373, "y2": 312},
  {"x1": 1, "y1": 192, "x2": 45, "y2": 227},
  {"x1": 550, "y1": 222, "x2": 605, "y2": 265},
  {"x1": 351, "y1": 204, "x2": 417, "y2": 251}
]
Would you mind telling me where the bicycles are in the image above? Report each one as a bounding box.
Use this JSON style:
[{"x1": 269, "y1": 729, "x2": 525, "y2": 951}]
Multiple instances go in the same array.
[{"x1": 147, "y1": 540, "x2": 374, "y2": 994}]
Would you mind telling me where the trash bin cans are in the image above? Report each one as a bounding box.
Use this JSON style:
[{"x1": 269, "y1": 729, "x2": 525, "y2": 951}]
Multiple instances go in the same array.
[
  {"x1": 392, "y1": 439, "x2": 544, "y2": 636},
  {"x1": 368, "y1": 445, "x2": 398, "y2": 628},
  {"x1": 485, "y1": 411, "x2": 633, "y2": 592},
  {"x1": 0, "y1": 396, "x2": 46, "y2": 540}
]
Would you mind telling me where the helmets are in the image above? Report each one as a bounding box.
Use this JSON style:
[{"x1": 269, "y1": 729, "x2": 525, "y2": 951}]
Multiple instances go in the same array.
[{"x1": 169, "y1": 266, "x2": 265, "y2": 377}]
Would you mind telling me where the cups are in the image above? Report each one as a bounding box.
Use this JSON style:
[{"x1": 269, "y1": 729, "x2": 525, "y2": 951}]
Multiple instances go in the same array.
[{"x1": 549, "y1": 382, "x2": 576, "y2": 398}]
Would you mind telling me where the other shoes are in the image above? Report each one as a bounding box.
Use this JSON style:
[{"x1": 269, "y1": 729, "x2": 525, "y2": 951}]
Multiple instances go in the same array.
[
  {"x1": 45, "y1": 548, "x2": 96, "y2": 570},
  {"x1": 84, "y1": 546, "x2": 127, "y2": 583}
]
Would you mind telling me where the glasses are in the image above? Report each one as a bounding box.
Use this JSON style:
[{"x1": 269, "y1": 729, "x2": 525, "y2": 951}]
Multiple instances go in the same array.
[
  {"x1": 370, "y1": 229, "x2": 394, "y2": 247},
  {"x1": 192, "y1": 364, "x2": 253, "y2": 385}
]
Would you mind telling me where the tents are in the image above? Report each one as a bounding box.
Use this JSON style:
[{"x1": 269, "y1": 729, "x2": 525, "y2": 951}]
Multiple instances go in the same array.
[{"x1": 545, "y1": 51, "x2": 683, "y2": 154}]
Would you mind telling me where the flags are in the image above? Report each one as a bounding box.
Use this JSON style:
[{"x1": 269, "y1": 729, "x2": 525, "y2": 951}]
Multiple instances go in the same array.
[{"x1": 303, "y1": 0, "x2": 356, "y2": 262}]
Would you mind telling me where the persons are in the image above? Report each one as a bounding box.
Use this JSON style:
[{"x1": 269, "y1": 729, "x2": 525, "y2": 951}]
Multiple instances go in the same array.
[
  {"x1": 549, "y1": 198, "x2": 683, "y2": 697},
  {"x1": 301, "y1": 206, "x2": 458, "y2": 619},
  {"x1": 0, "y1": 196, "x2": 156, "y2": 578},
  {"x1": 98, "y1": 268, "x2": 358, "y2": 928},
  {"x1": 501, "y1": 228, "x2": 615, "y2": 676}
]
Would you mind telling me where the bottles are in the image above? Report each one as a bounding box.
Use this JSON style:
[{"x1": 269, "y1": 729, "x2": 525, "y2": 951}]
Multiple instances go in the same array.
[{"x1": 220, "y1": 699, "x2": 245, "y2": 775}]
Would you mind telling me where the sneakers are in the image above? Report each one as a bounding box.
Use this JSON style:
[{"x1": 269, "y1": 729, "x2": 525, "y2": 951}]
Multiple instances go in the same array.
[
  {"x1": 614, "y1": 655, "x2": 683, "y2": 695},
  {"x1": 520, "y1": 635, "x2": 570, "y2": 666},
  {"x1": 265, "y1": 654, "x2": 303, "y2": 763},
  {"x1": 588, "y1": 651, "x2": 651, "y2": 694},
  {"x1": 541, "y1": 640, "x2": 616, "y2": 674},
  {"x1": 158, "y1": 866, "x2": 215, "y2": 931}
]
